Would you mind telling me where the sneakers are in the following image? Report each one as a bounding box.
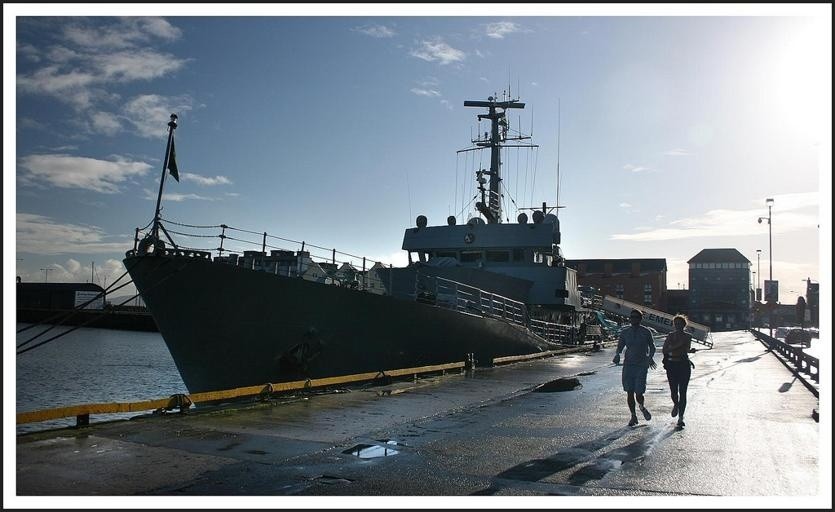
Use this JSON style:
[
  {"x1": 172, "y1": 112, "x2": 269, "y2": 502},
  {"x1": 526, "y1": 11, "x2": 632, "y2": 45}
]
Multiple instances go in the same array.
[{"x1": 627, "y1": 404, "x2": 687, "y2": 428}]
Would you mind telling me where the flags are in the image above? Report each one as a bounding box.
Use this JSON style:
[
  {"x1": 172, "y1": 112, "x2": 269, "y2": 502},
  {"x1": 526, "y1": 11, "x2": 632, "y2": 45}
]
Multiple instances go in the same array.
[{"x1": 167, "y1": 136, "x2": 180, "y2": 183}]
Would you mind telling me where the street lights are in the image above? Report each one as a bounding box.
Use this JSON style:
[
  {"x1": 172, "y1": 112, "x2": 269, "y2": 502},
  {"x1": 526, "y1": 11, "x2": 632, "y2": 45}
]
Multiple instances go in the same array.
[
  {"x1": 756, "y1": 250, "x2": 762, "y2": 333},
  {"x1": 757, "y1": 198, "x2": 778, "y2": 339}
]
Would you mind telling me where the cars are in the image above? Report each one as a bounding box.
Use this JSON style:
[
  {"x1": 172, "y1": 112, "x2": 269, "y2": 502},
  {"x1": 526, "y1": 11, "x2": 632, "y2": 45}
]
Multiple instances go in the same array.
[{"x1": 774, "y1": 326, "x2": 819, "y2": 348}]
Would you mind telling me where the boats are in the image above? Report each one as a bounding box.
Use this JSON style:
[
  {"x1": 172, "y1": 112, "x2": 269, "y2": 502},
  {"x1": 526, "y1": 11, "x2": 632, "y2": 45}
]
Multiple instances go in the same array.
[
  {"x1": 121, "y1": 67, "x2": 633, "y2": 409},
  {"x1": 16, "y1": 261, "x2": 160, "y2": 332}
]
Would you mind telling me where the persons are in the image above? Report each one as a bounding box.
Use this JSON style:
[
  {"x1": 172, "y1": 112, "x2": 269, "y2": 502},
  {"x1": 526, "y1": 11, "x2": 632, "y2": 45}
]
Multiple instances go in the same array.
[
  {"x1": 661, "y1": 313, "x2": 696, "y2": 426},
  {"x1": 612, "y1": 307, "x2": 658, "y2": 427}
]
